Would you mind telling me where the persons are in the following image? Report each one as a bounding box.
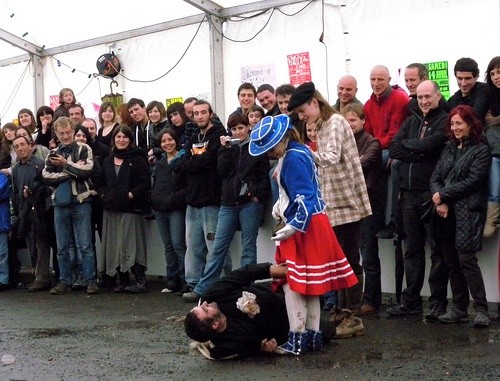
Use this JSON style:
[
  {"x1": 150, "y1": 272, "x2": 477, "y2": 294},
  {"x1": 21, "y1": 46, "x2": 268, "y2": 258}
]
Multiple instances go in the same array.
[
  {"x1": 287, "y1": 81, "x2": 373, "y2": 338},
  {"x1": 0, "y1": 56, "x2": 500, "y2": 330},
  {"x1": 184, "y1": 262, "x2": 337, "y2": 360},
  {"x1": 248, "y1": 113, "x2": 359, "y2": 356}
]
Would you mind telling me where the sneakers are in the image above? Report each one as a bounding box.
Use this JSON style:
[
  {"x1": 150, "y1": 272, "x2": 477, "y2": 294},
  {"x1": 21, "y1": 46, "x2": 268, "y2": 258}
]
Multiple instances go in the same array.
[
  {"x1": 438, "y1": 308, "x2": 467, "y2": 322},
  {"x1": 473, "y1": 312, "x2": 488, "y2": 326},
  {"x1": 335, "y1": 313, "x2": 364, "y2": 339},
  {"x1": 428, "y1": 305, "x2": 444, "y2": 319},
  {"x1": 387, "y1": 302, "x2": 422, "y2": 314}
]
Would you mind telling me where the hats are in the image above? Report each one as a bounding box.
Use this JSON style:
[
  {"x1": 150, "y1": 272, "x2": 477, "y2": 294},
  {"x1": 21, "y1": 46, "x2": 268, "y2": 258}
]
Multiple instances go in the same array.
[
  {"x1": 248, "y1": 114, "x2": 290, "y2": 156},
  {"x1": 287, "y1": 82, "x2": 315, "y2": 111}
]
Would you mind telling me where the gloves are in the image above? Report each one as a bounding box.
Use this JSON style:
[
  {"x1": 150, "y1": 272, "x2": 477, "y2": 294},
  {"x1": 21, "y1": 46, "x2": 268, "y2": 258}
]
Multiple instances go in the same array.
[
  {"x1": 272, "y1": 202, "x2": 282, "y2": 221},
  {"x1": 271, "y1": 226, "x2": 295, "y2": 241}
]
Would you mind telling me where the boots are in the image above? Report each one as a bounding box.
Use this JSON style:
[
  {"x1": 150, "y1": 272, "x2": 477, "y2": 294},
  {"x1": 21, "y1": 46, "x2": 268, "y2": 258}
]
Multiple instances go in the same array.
[
  {"x1": 482, "y1": 201, "x2": 499, "y2": 238},
  {"x1": 276, "y1": 330, "x2": 310, "y2": 355},
  {"x1": 304, "y1": 328, "x2": 323, "y2": 353},
  {"x1": 114, "y1": 270, "x2": 125, "y2": 291},
  {"x1": 124, "y1": 268, "x2": 150, "y2": 292}
]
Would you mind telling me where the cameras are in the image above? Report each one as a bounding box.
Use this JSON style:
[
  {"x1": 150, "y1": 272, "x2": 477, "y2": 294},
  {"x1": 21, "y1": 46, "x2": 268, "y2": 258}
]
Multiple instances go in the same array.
[{"x1": 225, "y1": 136, "x2": 240, "y2": 146}]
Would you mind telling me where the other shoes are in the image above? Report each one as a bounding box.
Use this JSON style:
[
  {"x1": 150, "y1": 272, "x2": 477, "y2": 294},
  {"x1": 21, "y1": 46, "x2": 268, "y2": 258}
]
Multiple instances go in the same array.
[
  {"x1": 0, "y1": 281, "x2": 21, "y2": 292},
  {"x1": 376, "y1": 225, "x2": 399, "y2": 240},
  {"x1": 161, "y1": 288, "x2": 174, "y2": 293},
  {"x1": 174, "y1": 287, "x2": 193, "y2": 296},
  {"x1": 87, "y1": 283, "x2": 99, "y2": 293},
  {"x1": 28, "y1": 281, "x2": 48, "y2": 292},
  {"x1": 73, "y1": 274, "x2": 84, "y2": 287},
  {"x1": 361, "y1": 304, "x2": 378, "y2": 313},
  {"x1": 182, "y1": 292, "x2": 200, "y2": 301},
  {"x1": 49, "y1": 283, "x2": 72, "y2": 294}
]
